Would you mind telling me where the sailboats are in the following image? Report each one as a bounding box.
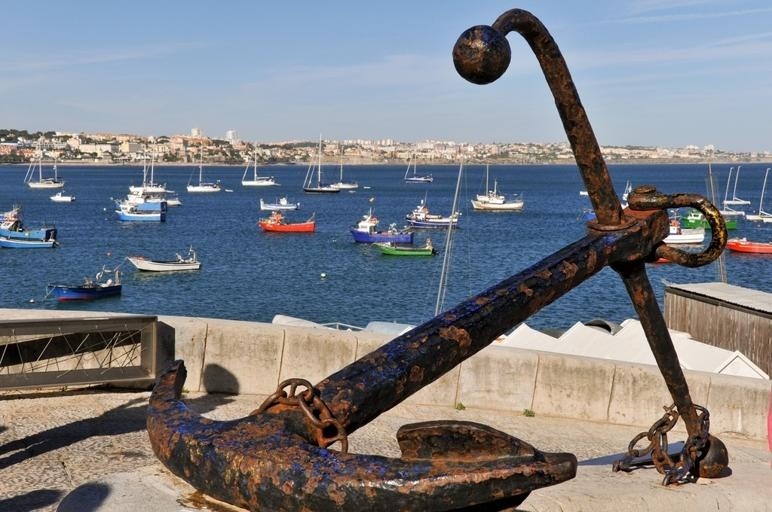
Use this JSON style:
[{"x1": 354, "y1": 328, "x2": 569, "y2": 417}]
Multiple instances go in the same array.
[
  {"x1": 241, "y1": 140, "x2": 282, "y2": 188},
  {"x1": 25, "y1": 136, "x2": 66, "y2": 189},
  {"x1": 185, "y1": 141, "x2": 222, "y2": 193},
  {"x1": 580, "y1": 160, "x2": 772, "y2": 264},
  {"x1": 330, "y1": 146, "x2": 358, "y2": 190},
  {"x1": 404, "y1": 150, "x2": 434, "y2": 185},
  {"x1": 129, "y1": 142, "x2": 175, "y2": 196},
  {"x1": 303, "y1": 135, "x2": 340, "y2": 193},
  {"x1": 477, "y1": 161, "x2": 506, "y2": 204}
]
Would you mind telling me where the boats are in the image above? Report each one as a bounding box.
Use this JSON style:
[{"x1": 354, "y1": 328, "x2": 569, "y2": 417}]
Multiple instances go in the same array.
[
  {"x1": 258, "y1": 211, "x2": 317, "y2": 233},
  {"x1": 115, "y1": 193, "x2": 184, "y2": 224},
  {"x1": 349, "y1": 190, "x2": 462, "y2": 257},
  {"x1": 46, "y1": 267, "x2": 124, "y2": 303},
  {"x1": 1, "y1": 205, "x2": 59, "y2": 250},
  {"x1": 50, "y1": 191, "x2": 76, "y2": 204},
  {"x1": 126, "y1": 245, "x2": 202, "y2": 272},
  {"x1": 470, "y1": 200, "x2": 524, "y2": 211},
  {"x1": 257, "y1": 195, "x2": 304, "y2": 213}
]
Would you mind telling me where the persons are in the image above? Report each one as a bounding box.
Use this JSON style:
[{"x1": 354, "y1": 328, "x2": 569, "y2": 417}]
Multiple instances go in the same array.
[{"x1": 426, "y1": 238, "x2": 434, "y2": 250}]
